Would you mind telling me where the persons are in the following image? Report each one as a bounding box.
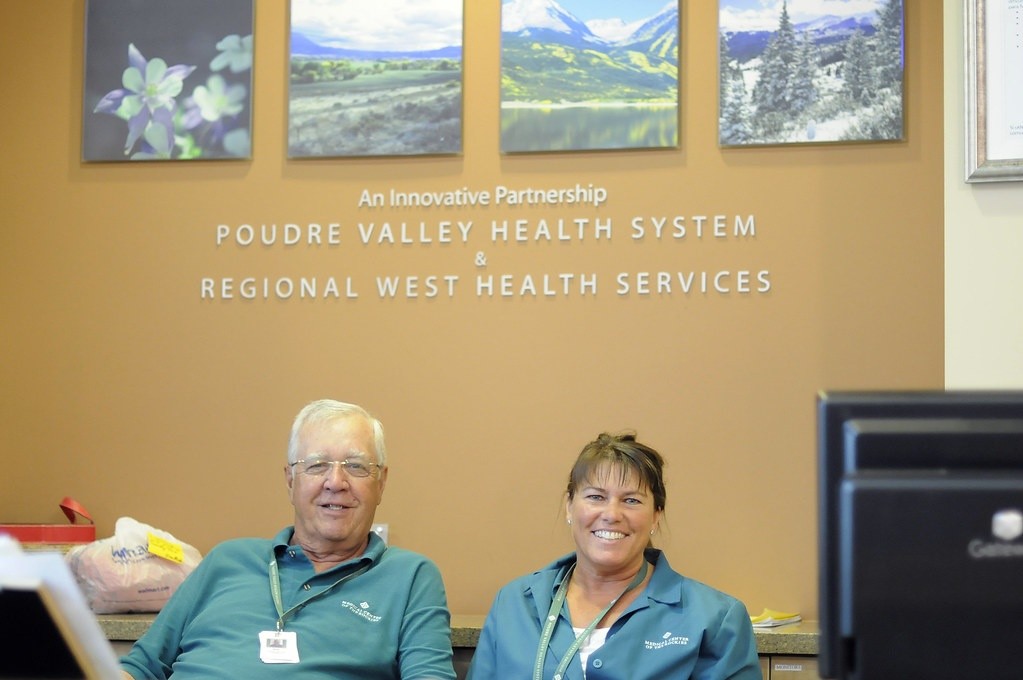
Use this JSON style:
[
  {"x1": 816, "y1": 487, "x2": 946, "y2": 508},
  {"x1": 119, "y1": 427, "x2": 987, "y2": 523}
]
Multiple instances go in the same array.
[
  {"x1": 116, "y1": 398, "x2": 458, "y2": 680},
  {"x1": 465, "y1": 434, "x2": 765, "y2": 680}
]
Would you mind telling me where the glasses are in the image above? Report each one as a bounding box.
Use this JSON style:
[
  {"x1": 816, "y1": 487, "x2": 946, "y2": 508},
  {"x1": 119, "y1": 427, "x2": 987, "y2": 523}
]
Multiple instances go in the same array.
[{"x1": 291, "y1": 457, "x2": 382, "y2": 477}]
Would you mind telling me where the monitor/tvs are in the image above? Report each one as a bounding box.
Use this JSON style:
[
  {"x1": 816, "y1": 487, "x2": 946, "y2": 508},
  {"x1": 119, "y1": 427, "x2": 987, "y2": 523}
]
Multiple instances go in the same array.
[{"x1": 816, "y1": 389, "x2": 1023, "y2": 680}]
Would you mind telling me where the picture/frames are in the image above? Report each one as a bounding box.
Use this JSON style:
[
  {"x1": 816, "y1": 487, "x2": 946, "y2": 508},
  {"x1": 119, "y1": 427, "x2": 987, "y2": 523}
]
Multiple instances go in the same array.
[{"x1": 964, "y1": 0, "x2": 1023, "y2": 184}]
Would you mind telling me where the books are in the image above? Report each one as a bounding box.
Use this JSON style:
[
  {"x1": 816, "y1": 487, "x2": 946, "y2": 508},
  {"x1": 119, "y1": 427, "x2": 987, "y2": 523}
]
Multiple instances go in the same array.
[{"x1": 751, "y1": 608, "x2": 801, "y2": 628}]
